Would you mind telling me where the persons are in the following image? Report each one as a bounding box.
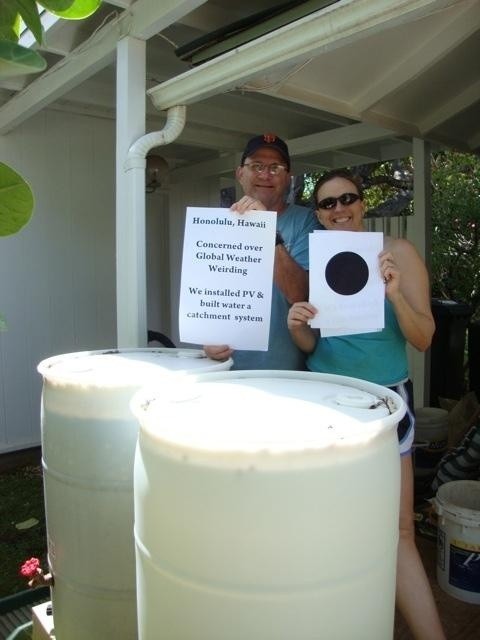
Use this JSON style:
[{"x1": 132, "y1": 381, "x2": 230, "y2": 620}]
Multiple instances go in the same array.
[
  {"x1": 203, "y1": 134, "x2": 319, "y2": 373},
  {"x1": 287, "y1": 167, "x2": 449, "y2": 639}
]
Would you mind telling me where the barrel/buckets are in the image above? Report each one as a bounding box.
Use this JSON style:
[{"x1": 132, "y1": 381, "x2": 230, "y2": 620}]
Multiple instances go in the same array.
[
  {"x1": 37, "y1": 347, "x2": 233, "y2": 640},
  {"x1": 133, "y1": 370, "x2": 406, "y2": 640},
  {"x1": 423, "y1": 480, "x2": 480, "y2": 604},
  {"x1": 413, "y1": 407, "x2": 449, "y2": 478}
]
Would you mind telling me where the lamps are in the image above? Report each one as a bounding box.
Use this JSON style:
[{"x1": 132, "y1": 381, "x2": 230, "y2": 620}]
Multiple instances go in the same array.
[{"x1": 146, "y1": 154, "x2": 170, "y2": 194}]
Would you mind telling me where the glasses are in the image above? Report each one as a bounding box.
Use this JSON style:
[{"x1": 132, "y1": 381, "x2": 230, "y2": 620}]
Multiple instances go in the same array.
[
  {"x1": 317, "y1": 192, "x2": 363, "y2": 209},
  {"x1": 240, "y1": 159, "x2": 288, "y2": 174}
]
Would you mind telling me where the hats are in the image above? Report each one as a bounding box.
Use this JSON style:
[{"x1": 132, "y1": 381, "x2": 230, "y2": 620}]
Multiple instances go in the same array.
[{"x1": 240, "y1": 134, "x2": 289, "y2": 157}]
